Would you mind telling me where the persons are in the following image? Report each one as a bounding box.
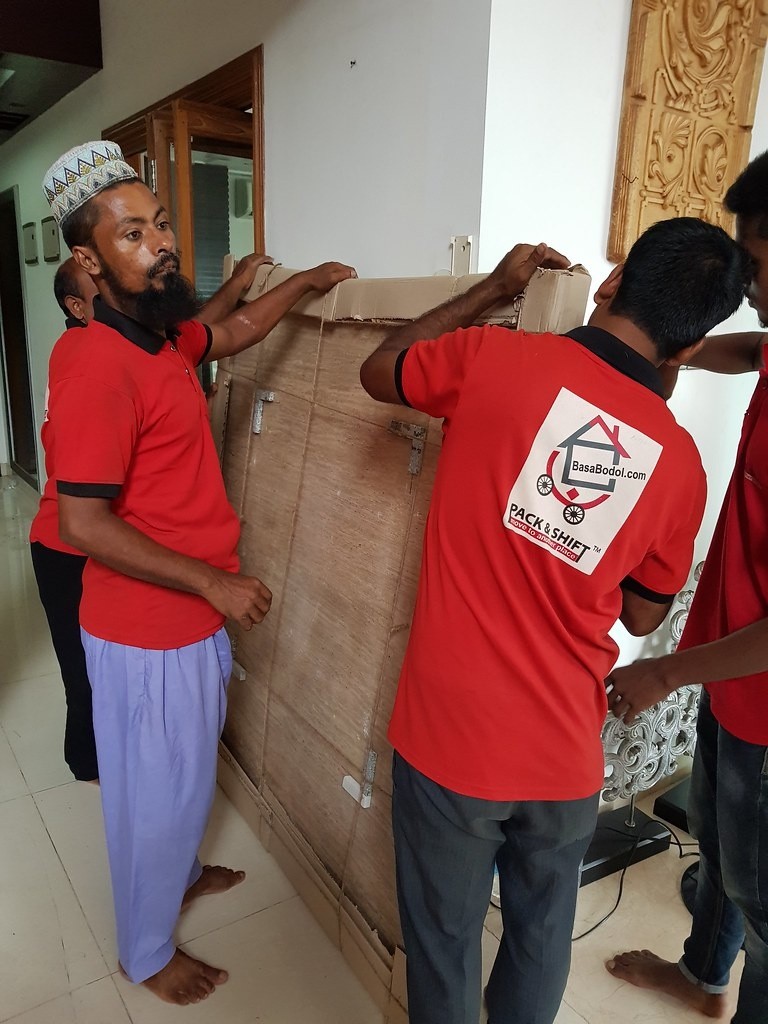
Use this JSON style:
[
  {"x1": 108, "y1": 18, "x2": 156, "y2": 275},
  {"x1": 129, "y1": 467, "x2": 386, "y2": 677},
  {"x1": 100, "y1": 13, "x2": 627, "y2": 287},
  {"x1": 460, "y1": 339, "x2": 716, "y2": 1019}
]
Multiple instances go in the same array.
[
  {"x1": 358, "y1": 216, "x2": 754, "y2": 1024},
  {"x1": 595, "y1": 151, "x2": 768, "y2": 1024},
  {"x1": 31, "y1": 255, "x2": 277, "y2": 784},
  {"x1": 50, "y1": 168, "x2": 355, "y2": 1005}
]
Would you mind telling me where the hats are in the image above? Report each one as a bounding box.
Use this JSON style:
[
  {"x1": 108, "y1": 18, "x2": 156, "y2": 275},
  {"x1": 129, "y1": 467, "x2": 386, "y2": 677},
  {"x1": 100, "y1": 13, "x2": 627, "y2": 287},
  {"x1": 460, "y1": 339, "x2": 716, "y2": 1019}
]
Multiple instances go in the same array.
[{"x1": 42, "y1": 140, "x2": 138, "y2": 227}]
